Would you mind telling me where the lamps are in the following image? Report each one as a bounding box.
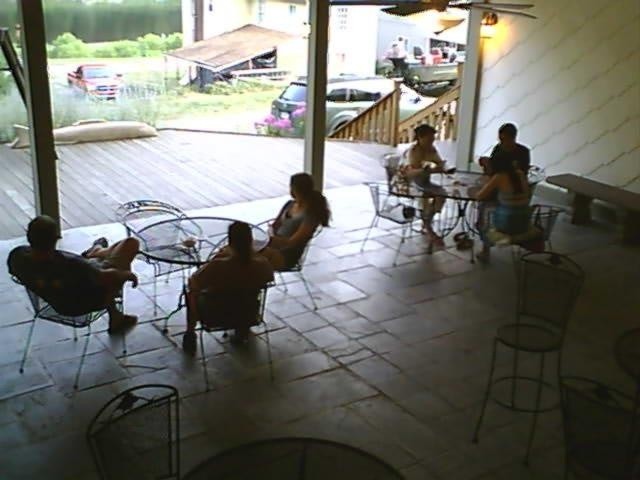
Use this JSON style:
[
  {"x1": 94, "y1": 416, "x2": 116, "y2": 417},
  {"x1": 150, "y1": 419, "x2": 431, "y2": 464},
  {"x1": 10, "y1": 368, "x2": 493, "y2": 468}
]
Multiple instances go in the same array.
[{"x1": 479, "y1": 7, "x2": 498, "y2": 38}]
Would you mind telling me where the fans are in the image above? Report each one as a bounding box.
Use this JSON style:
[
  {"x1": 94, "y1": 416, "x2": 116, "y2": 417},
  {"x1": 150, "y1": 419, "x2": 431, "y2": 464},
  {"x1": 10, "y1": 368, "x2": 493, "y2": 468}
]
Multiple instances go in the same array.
[{"x1": 380, "y1": 0, "x2": 539, "y2": 19}]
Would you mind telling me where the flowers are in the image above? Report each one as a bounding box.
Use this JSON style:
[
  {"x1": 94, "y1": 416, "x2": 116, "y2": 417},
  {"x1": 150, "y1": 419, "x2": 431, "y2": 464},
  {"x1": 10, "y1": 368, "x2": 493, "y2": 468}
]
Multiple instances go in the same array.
[{"x1": 264, "y1": 99, "x2": 308, "y2": 138}]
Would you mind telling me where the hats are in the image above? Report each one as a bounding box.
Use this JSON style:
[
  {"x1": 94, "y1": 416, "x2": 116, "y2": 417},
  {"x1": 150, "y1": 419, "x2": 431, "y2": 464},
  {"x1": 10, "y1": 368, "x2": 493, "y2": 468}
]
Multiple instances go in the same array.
[{"x1": 414, "y1": 125, "x2": 439, "y2": 135}]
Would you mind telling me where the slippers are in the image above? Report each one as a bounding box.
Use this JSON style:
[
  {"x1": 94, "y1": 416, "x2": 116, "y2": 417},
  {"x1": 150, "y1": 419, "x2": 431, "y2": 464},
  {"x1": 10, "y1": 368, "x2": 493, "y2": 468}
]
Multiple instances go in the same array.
[
  {"x1": 83, "y1": 236, "x2": 108, "y2": 260},
  {"x1": 108, "y1": 314, "x2": 138, "y2": 330}
]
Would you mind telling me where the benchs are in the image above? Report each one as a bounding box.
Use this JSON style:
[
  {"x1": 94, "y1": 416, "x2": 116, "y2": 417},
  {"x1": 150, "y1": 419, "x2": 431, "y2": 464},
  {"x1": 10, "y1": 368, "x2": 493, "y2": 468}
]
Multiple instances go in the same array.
[{"x1": 546, "y1": 173, "x2": 640, "y2": 247}]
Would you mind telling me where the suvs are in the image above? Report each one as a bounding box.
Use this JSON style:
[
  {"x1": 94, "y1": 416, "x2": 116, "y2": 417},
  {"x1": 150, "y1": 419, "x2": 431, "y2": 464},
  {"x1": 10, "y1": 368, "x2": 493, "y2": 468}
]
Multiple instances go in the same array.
[{"x1": 272, "y1": 78, "x2": 425, "y2": 138}]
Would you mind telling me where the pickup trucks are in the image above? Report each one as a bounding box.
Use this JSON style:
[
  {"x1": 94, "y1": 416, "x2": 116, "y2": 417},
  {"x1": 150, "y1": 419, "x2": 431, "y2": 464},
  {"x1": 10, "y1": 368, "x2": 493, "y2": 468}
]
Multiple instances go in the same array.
[{"x1": 68, "y1": 63, "x2": 125, "y2": 98}]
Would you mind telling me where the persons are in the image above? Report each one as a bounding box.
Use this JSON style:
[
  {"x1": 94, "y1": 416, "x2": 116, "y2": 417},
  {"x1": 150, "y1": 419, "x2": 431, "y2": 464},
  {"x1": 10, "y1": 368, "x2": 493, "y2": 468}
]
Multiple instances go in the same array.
[
  {"x1": 181, "y1": 219, "x2": 274, "y2": 356},
  {"x1": 259, "y1": 173, "x2": 332, "y2": 271},
  {"x1": 463, "y1": 148, "x2": 531, "y2": 265},
  {"x1": 387, "y1": 37, "x2": 458, "y2": 67},
  {"x1": 7, "y1": 212, "x2": 140, "y2": 337},
  {"x1": 482, "y1": 123, "x2": 530, "y2": 181},
  {"x1": 395, "y1": 124, "x2": 446, "y2": 249}
]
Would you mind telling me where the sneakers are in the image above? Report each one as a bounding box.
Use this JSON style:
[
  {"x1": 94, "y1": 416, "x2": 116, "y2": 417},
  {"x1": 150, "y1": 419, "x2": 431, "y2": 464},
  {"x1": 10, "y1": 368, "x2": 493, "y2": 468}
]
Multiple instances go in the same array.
[{"x1": 181, "y1": 330, "x2": 198, "y2": 349}]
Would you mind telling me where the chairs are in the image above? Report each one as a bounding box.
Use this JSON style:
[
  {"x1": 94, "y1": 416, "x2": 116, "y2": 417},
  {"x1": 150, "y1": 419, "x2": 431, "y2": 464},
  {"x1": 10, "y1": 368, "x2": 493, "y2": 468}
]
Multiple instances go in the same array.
[
  {"x1": 84, "y1": 383, "x2": 179, "y2": 480},
  {"x1": 375, "y1": 159, "x2": 564, "y2": 280},
  {"x1": 470, "y1": 250, "x2": 586, "y2": 466},
  {"x1": 560, "y1": 376, "x2": 640, "y2": 480}
]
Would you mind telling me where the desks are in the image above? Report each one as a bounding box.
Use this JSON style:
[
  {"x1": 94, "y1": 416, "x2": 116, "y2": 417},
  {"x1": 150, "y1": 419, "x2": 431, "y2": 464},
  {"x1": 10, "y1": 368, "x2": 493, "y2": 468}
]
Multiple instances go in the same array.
[
  {"x1": 613, "y1": 327, "x2": 640, "y2": 382},
  {"x1": 181, "y1": 436, "x2": 409, "y2": 479}
]
